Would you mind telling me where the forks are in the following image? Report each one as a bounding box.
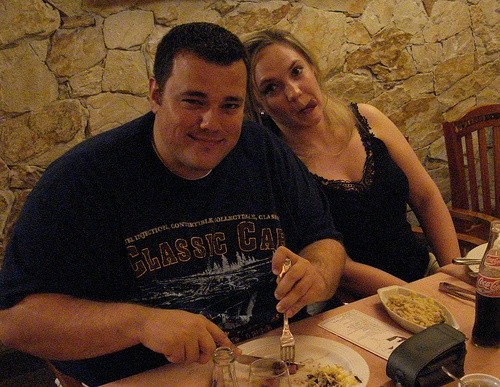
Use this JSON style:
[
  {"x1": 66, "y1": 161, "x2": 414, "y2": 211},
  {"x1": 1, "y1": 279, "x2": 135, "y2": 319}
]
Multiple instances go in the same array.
[{"x1": 279, "y1": 259, "x2": 296, "y2": 365}]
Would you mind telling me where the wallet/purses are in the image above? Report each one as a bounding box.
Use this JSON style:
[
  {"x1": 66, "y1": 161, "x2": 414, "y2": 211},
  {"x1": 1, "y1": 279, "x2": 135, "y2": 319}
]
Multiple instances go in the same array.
[{"x1": 386, "y1": 323, "x2": 466, "y2": 387}]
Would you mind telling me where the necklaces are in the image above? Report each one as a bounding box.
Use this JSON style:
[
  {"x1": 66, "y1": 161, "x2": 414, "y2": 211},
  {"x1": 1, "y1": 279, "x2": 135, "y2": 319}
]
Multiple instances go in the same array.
[{"x1": 290, "y1": 133, "x2": 351, "y2": 158}]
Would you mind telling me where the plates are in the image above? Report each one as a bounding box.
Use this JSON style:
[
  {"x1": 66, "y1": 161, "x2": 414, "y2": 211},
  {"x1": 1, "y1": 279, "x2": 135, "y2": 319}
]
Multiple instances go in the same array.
[
  {"x1": 377, "y1": 285, "x2": 459, "y2": 335},
  {"x1": 236, "y1": 334, "x2": 370, "y2": 387},
  {"x1": 467, "y1": 242, "x2": 488, "y2": 272}
]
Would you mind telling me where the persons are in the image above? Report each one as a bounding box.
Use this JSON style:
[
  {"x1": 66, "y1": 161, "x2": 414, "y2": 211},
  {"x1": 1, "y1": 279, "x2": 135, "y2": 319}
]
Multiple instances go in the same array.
[
  {"x1": 242, "y1": 28, "x2": 463, "y2": 316},
  {"x1": 1, "y1": 21, "x2": 345, "y2": 387}
]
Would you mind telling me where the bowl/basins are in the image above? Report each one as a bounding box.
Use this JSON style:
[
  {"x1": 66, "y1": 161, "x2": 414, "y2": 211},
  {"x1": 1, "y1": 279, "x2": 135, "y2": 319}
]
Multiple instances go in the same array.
[{"x1": 457, "y1": 373, "x2": 500, "y2": 387}]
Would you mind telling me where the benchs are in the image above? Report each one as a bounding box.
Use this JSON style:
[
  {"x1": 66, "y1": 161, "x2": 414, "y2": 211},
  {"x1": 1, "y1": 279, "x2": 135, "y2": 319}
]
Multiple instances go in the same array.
[{"x1": 43, "y1": 209, "x2": 500, "y2": 387}]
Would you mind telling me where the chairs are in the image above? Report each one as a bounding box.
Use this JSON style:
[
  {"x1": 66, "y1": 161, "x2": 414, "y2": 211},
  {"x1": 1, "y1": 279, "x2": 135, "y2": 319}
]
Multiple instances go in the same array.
[{"x1": 442, "y1": 104, "x2": 500, "y2": 258}]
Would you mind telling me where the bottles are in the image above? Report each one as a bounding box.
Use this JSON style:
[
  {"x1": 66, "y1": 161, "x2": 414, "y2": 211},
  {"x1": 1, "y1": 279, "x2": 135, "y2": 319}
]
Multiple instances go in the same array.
[
  {"x1": 472, "y1": 220, "x2": 500, "y2": 348},
  {"x1": 211, "y1": 347, "x2": 238, "y2": 387}
]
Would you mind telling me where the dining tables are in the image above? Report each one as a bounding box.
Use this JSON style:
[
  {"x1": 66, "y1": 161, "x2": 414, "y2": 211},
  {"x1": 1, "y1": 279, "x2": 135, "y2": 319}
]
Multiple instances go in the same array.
[{"x1": 97, "y1": 273, "x2": 500, "y2": 387}]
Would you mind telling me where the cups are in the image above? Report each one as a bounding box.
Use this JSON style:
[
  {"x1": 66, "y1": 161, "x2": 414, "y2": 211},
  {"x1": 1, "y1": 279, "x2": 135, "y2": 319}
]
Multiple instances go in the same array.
[{"x1": 248, "y1": 358, "x2": 291, "y2": 387}]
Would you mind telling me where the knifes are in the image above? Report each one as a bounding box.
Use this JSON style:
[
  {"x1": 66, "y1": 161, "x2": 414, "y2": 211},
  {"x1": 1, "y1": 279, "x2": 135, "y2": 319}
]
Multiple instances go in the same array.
[{"x1": 233, "y1": 353, "x2": 305, "y2": 375}]
